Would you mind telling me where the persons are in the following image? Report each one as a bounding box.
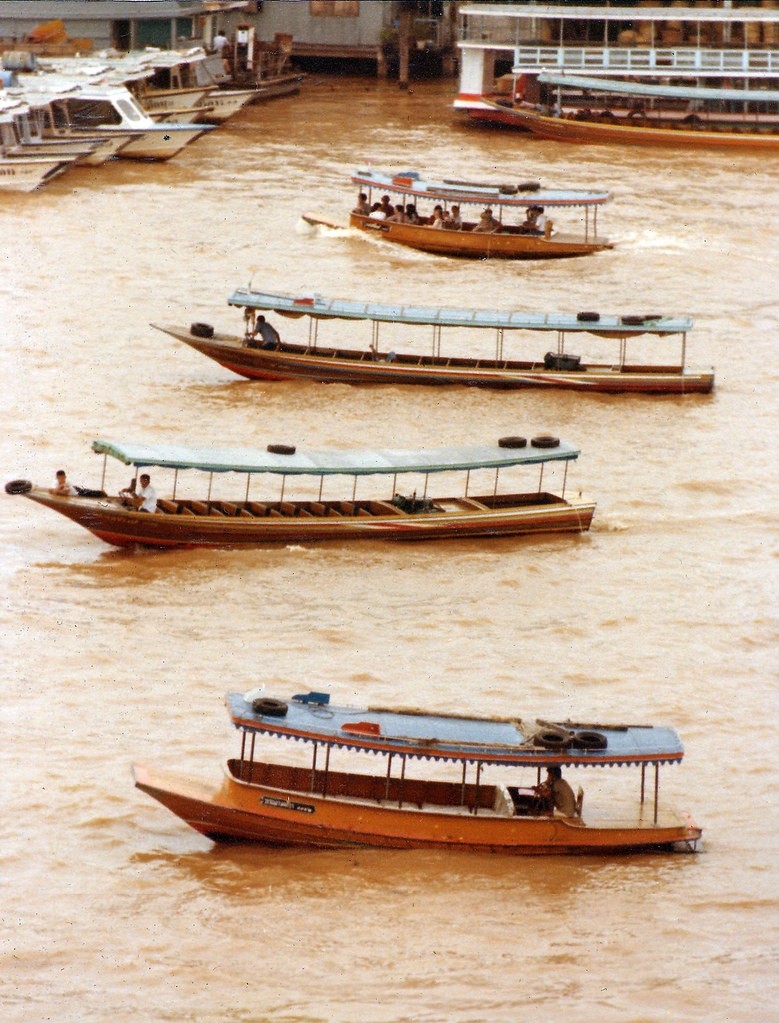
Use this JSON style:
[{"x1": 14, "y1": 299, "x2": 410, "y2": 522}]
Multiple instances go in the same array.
[
  {"x1": 245, "y1": 315, "x2": 281, "y2": 351},
  {"x1": 515, "y1": 88, "x2": 561, "y2": 118},
  {"x1": 202, "y1": 29, "x2": 233, "y2": 59},
  {"x1": 121, "y1": 474, "x2": 157, "y2": 513},
  {"x1": 49, "y1": 471, "x2": 107, "y2": 498},
  {"x1": 532, "y1": 765, "x2": 576, "y2": 819},
  {"x1": 354, "y1": 193, "x2": 548, "y2": 236}
]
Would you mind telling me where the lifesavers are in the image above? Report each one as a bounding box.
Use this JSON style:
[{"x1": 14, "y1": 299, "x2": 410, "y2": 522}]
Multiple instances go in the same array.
[
  {"x1": 190, "y1": 321, "x2": 214, "y2": 338},
  {"x1": 498, "y1": 181, "x2": 541, "y2": 194},
  {"x1": 266, "y1": 443, "x2": 296, "y2": 455},
  {"x1": 621, "y1": 316, "x2": 644, "y2": 325},
  {"x1": 577, "y1": 311, "x2": 600, "y2": 321},
  {"x1": 499, "y1": 436, "x2": 527, "y2": 448},
  {"x1": 533, "y1": 729, "x2": 609, "y2": 749},
  {"x1": 251, "y1": 696, "x2": 288, "y2": 718},
  {"x1": 531, "y1": 435, "x2": 560, "y2": 449}
]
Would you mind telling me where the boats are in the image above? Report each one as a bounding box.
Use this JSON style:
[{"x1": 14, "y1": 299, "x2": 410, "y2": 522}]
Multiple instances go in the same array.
[
  {"x1": 453, "y1": 3, "x2": 779, "y2": 127},
  {"x1": 132, "y1": 685, "x2": 705, "y2": 851},
  {"x1": 299, "y1": 163, "x2": 615, "y2": 257},
  {"x1": 0, "y1": 45, "x2": 308, "y2": 194},
  {"x1": 148, "y1": 287, "x2": 713, "y2": 394},
  {"x1": 478, "y1": 71, "x2": 779, "y2": 148},
  {"x1": 5, "y1": 440, "x2": 599, "y2": 548}
]
